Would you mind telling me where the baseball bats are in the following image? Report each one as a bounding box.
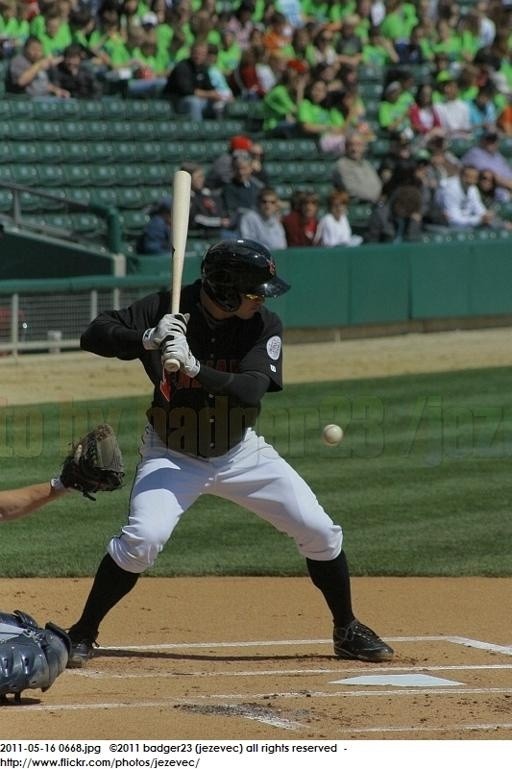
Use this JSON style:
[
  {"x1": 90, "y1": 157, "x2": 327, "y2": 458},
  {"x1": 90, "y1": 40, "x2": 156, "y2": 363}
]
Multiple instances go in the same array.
[{"x1": 164, "y1": 171, "x2": 192, "y2": 373}]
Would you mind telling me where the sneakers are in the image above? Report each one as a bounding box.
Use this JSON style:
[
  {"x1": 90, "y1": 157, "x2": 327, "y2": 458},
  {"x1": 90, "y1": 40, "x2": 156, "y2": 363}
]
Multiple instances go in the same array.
[
  {"x1": 333, "y1": 618, "x2": 393, "y2": 661},
  {"x1": 66, "y1": 637, "x2": 94, "y2": 666}
]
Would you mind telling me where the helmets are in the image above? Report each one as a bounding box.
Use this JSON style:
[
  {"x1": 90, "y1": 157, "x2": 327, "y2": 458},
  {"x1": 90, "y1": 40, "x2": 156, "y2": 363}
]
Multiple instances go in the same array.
[{"x1": 202, "y1": 239, "x2": 291, "y2": 297}]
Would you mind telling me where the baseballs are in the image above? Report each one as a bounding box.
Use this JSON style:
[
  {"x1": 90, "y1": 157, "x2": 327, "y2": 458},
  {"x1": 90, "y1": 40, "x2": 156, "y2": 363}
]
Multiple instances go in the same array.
[{"x1": 323, "y1": 424, "x2": 343, "y2": 446}]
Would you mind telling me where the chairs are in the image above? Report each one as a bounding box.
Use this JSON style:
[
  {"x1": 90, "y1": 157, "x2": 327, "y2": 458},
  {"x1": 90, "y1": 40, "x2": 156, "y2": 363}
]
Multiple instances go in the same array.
[{"x1": 0, "y1": 1, "x2": 512, "y2": 265}]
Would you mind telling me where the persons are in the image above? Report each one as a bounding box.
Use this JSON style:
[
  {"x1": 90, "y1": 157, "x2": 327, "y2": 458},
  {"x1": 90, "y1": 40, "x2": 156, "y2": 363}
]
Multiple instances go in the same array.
[
  {"x1": 67, "y1": 237, "x2": 394, "y2": 669},
  {"x1": 0, "y1": 426, "x2": 125, "y2": 698}
]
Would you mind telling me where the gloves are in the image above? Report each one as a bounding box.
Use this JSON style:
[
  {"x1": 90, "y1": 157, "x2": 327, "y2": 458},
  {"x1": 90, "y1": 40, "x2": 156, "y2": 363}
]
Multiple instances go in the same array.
[{"x1": 142, "y1": 313, "x2": 201, "y2": 378}]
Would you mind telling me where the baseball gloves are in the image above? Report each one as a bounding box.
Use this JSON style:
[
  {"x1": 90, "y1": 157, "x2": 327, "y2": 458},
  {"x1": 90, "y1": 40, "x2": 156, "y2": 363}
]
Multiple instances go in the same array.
[{"x1": 60, "y1": 423, "x2": 125, "y2": 501}]
